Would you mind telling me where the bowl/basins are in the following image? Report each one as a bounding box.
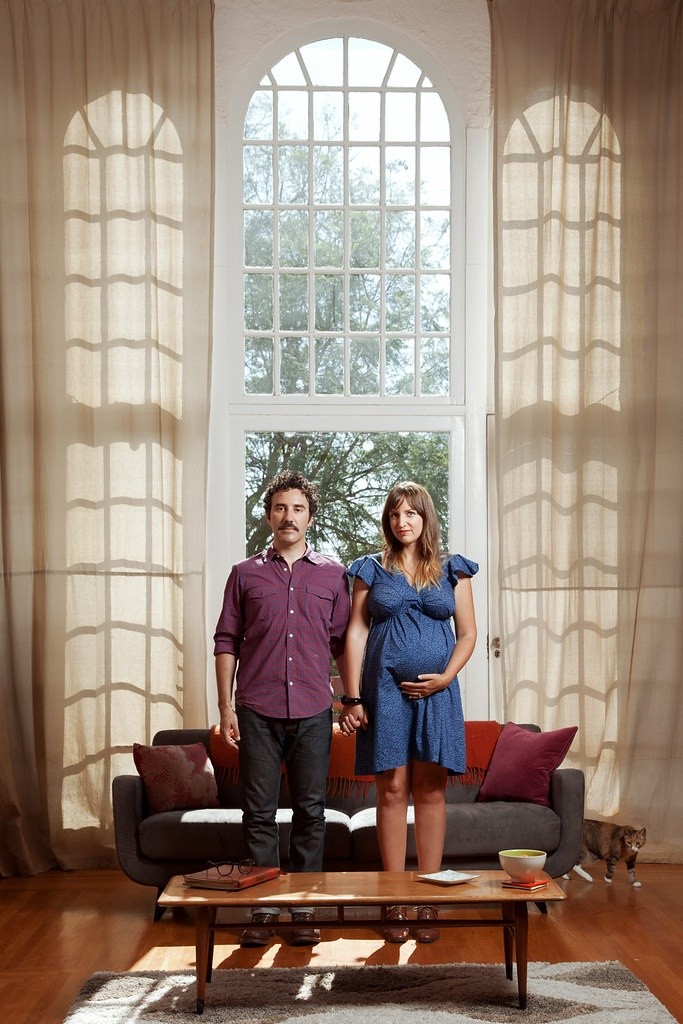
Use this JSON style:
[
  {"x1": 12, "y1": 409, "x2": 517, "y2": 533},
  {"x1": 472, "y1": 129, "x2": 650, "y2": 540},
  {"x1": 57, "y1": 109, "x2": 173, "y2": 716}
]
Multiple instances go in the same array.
[{"x1": 498, "y1": 849, "x2": 547, "y2": 883}]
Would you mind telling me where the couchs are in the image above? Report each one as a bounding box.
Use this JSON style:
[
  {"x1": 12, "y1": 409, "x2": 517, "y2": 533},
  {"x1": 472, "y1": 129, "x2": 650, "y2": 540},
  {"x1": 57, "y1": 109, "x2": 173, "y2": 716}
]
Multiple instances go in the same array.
[{"x1": 112, "y1": 721, "x2": 585, "y2": 923}]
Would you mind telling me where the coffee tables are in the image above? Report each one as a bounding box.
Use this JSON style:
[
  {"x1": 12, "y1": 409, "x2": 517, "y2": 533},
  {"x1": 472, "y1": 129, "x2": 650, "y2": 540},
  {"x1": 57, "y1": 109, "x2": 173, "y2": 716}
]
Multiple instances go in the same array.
[{"x1": 158, "y1": 871, "x2": 567, "y2": 1015}]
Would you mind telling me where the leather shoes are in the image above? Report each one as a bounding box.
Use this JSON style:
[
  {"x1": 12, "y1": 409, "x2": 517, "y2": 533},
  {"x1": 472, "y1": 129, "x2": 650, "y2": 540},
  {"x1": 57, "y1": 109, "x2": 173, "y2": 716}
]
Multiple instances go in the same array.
[
  {"x1": 289, "y1": 911, "x2": 323, "y2": 943},
  {"x1": 239, "y1": 914, "x2": 279, "y2": 947},
  {"x1": 381, "y1": 906, "x2": 411, "y2": 944},
  {"x1": 416, "y1": 906, "x2": 442, "y2": 944}
]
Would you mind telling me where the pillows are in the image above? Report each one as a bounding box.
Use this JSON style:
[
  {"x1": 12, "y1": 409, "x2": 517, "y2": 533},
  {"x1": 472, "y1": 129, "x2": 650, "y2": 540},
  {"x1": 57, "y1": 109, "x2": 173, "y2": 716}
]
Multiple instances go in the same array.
[
  {"x1": 477, "y1": 722, "x2": 579, "y2": 806},
  {"x1": 133, "y1": 743, "x2": 220, "y2": 814}
]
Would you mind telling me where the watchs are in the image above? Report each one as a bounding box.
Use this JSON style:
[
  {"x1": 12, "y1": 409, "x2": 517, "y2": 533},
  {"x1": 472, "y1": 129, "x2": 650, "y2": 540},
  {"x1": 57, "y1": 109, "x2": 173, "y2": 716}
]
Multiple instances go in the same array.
[{"x1": 340, "y1": 695, "x2": 363, "y2": 706}]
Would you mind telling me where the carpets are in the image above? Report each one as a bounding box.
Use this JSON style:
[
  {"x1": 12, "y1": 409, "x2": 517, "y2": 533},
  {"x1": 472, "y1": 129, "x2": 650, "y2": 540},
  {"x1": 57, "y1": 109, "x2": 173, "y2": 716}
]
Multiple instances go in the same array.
[{"x1": 64, "y1": 959, "x2": 680, "y2": 1024}]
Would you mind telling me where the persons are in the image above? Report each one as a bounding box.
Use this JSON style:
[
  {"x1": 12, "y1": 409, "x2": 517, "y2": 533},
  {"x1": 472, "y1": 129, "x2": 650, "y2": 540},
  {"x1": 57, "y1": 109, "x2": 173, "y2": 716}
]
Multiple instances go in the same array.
[
  {"x1": 339, "y1": 480, "x2": 480, "y2": 942},
  {"x1": 214, "y1": 470, "x2": 366, "y2": 947}
]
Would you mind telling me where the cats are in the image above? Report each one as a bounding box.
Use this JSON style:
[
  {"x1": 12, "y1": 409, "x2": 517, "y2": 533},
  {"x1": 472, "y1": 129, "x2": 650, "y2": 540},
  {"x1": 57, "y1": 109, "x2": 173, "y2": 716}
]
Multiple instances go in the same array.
[{"x1": 562, "y1": 819, "x2": 646, "y2": 888}]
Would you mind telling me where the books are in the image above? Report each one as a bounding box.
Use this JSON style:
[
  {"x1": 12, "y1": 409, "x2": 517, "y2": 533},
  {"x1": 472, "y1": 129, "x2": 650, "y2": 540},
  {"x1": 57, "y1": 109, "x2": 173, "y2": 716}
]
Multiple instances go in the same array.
[{"x1": 183, "y1": 865, "x2": 281, "y2": 891}]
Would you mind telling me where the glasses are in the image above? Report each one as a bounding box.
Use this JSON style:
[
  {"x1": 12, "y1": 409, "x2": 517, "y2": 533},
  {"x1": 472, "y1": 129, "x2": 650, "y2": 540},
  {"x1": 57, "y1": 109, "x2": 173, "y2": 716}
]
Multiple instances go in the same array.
[{"x1": 203, "y1": 856, "x2": 258, "y2": 875}]
